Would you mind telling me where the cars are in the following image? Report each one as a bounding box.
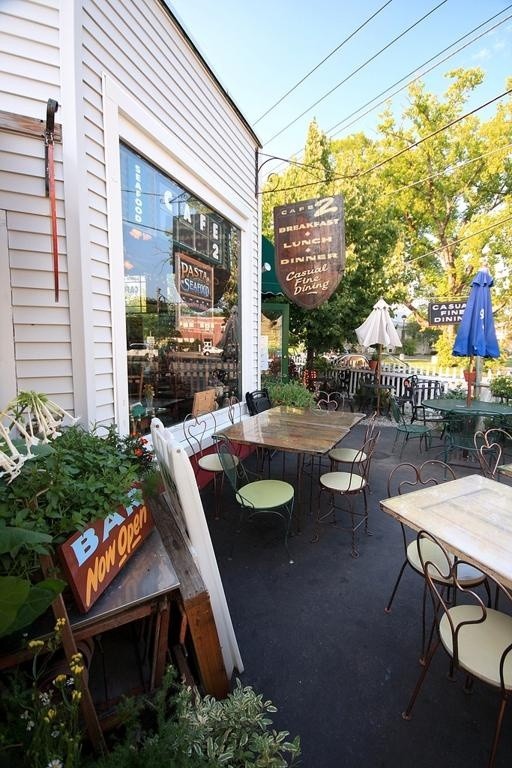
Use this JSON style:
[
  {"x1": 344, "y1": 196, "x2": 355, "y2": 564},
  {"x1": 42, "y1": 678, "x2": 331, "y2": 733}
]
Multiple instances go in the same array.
[
  {"x1": 127, "y1": 342, "x2": 158, "y2": 360},
  {"x1": 200, "y1": 346, "x2": 223, "y2": 356},
  {"x1": 329, "y1": 349, "x2": 447, "y2": 405}
]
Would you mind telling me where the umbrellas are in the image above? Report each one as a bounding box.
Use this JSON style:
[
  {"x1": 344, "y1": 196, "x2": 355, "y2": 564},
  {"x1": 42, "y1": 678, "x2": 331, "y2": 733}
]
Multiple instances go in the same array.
[
  {"x1": 354, "y1": 295, "x2": 403, "y2": 415},
  {"x1": 215, "y1": 312, "x2": 241, "y2": 389},
  {"x1": 452, "y1": 265, "x2": 499, "y2": 443}
]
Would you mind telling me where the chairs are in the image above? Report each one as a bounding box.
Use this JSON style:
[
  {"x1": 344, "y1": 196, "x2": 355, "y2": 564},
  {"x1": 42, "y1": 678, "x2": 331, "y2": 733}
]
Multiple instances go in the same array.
[
  {"x1": 128, "y1": 363, "x2": 183, "y2": 462},
  {"x1": 182, "y1": 364, "x2": 375, "y2": 566},
  {"x1": 375, "y1": 374, "x2": 512, "y2": 767}
]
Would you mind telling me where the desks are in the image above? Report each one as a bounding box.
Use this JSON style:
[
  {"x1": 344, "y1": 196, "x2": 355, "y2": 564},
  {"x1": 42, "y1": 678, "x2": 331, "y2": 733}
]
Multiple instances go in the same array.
[{"x1": 0, "y1": 507, "x2": 184, "y2": 767}]
